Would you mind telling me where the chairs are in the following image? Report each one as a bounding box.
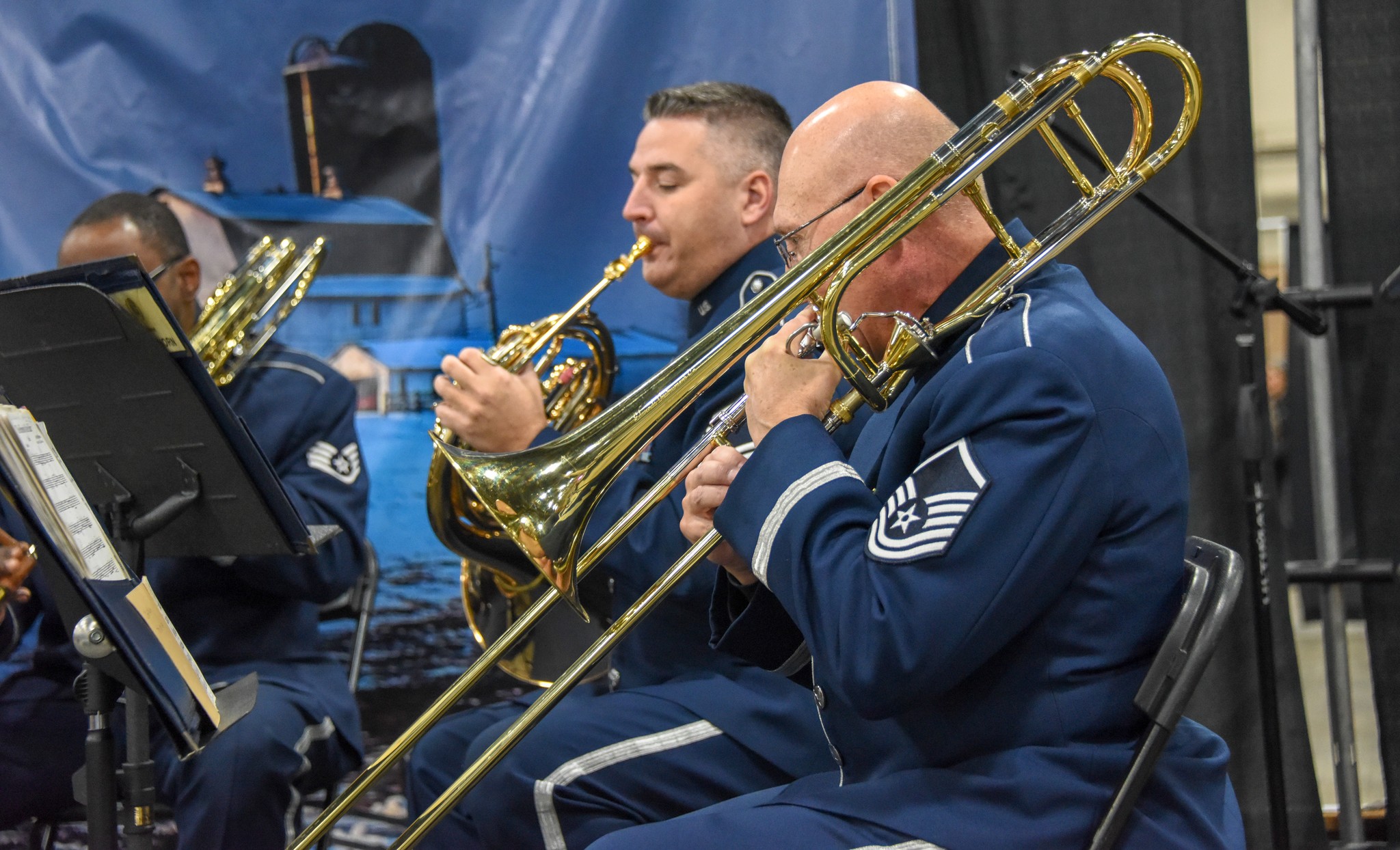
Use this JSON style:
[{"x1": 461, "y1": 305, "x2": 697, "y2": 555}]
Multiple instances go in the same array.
[{"x1": 1091, "y1": 530, "x2": 1242, "y2": 850}]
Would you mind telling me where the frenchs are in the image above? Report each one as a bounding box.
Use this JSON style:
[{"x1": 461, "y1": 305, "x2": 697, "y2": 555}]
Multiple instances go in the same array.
[{"x1": 426, "y1": 234, "x2": 657, "y2": 684}]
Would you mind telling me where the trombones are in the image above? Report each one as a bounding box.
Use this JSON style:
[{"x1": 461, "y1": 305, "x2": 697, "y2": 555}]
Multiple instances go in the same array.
[
  {"x1": 290, "y1": 28, "x2": 1203, "y2": 848},
  {"x1": 1, "y1": 232, "x2": 329, "y2": 602}
]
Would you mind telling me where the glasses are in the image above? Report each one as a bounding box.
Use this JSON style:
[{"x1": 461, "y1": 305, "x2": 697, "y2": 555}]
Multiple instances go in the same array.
[{"x1": 776, "y1": 184, "x2": 867, "y2": 266}]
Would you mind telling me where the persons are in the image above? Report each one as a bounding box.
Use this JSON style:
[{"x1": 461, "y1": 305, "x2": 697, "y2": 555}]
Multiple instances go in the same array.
[
  {"x1": 398, "y1": 76, "x2": 856, "y2": 849},
  {"x1": 582, "y1": 73, "x2": 1251, "y2": 850},
  {"x1": 0, "y1": 188, "x2": 382, "y2": 850}
]
